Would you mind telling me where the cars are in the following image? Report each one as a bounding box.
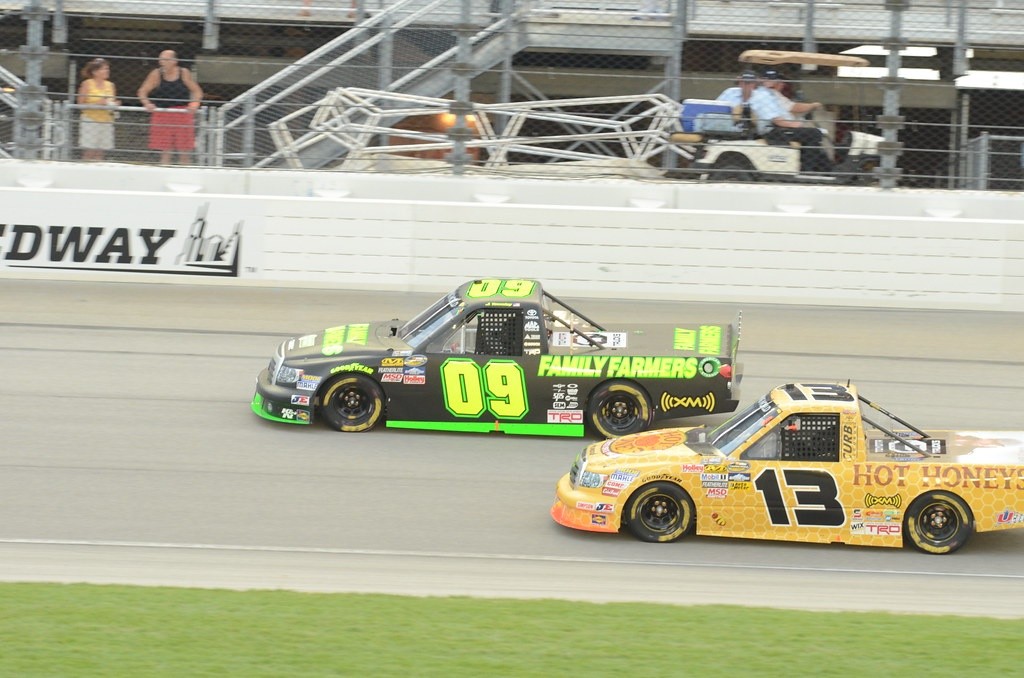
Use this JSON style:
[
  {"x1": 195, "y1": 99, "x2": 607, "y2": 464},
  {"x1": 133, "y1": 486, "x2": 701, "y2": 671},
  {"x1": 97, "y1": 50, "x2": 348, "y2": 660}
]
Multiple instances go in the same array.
[
  {"x1": 252, "y1": 276, "x2": 745, "y2": 441},
  {"x1": 550, "y1": 378, "x2": 1024, "y2": 554}
]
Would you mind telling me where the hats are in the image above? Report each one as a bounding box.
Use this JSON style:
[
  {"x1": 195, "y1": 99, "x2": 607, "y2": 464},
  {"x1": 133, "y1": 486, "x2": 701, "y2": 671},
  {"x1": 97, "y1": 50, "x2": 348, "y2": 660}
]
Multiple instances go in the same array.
[{"x1": 740, "y1": 70, "x2": 793, "y2": 82}]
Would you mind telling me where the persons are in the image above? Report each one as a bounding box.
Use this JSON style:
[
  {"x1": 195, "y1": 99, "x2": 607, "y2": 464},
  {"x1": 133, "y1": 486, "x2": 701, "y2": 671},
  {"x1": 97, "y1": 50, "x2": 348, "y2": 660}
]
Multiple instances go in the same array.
[
  {"x1": 747, "y1": 430, "x2": 777, "y2": 459},
  {"x1": 76, "y1": 60, "x2": 120, "y2": 163},
  {"x1": 714, "y1": 69, "x2": 833, "y2": 172},
  {"x1": 137, "y1": 50, "x2": 203, "y2": 164}
]
